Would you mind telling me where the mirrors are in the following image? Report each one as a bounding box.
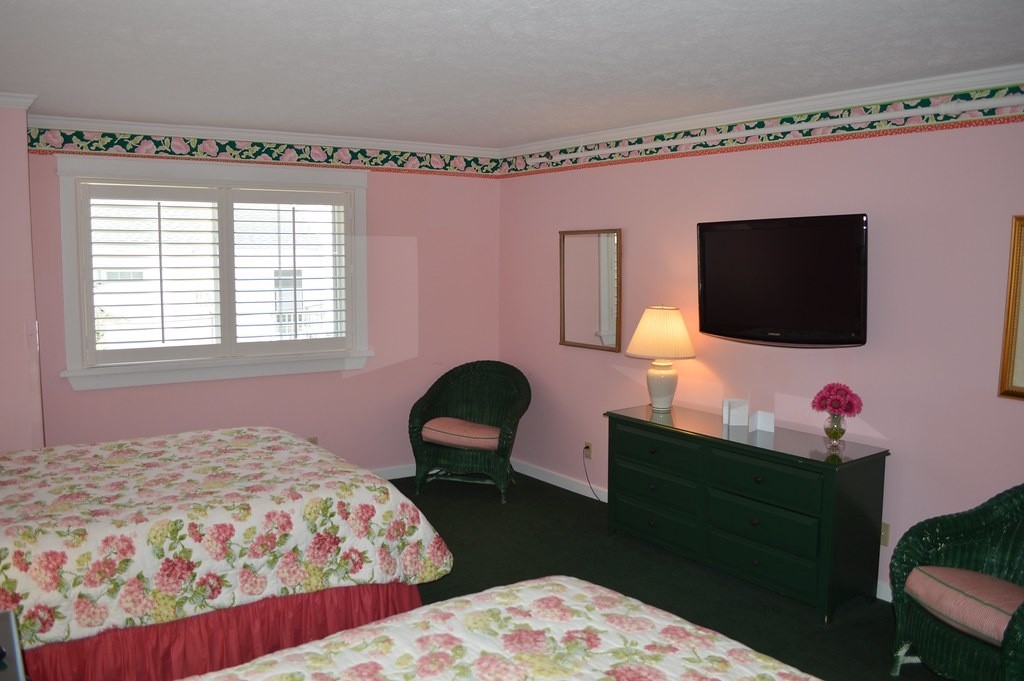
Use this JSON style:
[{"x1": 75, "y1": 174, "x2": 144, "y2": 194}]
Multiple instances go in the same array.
[{"x1": 559, "y1": 228, "x2": 622, "y2": 354}]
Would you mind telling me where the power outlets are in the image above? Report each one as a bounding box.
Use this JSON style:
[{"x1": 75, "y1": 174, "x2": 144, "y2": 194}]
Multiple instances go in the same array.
[
  {"x1": 880, "y1": 523, "x2": 890, "y2": 547},
  {"x1": 584, "y1": 441, "x2": 592, "y2": 459}
]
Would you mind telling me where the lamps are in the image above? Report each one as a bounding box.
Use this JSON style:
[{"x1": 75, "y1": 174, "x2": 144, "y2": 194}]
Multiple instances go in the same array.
[
  {"x1": 650, "y1": 409, "x2": 674, "y2": 427},
  {"x1": 625, "y1": 303, "x2": 697, "y2": 410}
]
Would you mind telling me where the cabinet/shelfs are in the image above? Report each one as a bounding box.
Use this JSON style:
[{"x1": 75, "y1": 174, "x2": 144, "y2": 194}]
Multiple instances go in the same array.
[{"x1": 602, "y1": 405, "x2": 890, "y2": 626}]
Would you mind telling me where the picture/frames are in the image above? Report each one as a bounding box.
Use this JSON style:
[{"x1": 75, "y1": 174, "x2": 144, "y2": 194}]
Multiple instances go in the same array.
[{"x1": 996, "y1": 215, "x2": 1024, "y2": 401}]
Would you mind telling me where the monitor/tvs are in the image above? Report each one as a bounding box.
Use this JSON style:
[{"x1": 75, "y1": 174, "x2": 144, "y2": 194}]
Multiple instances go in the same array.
[{"x1": 697, "y1": 214, "x2": 867, "y2": 347}]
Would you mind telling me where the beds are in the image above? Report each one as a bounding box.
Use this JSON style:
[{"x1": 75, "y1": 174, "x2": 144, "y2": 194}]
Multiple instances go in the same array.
[
  {"x1": 178, "y1": 575, "x2": 826, "y2": 681},
  {"x1": 0, "y1": 426, "x2": 455, "y2": 681}
]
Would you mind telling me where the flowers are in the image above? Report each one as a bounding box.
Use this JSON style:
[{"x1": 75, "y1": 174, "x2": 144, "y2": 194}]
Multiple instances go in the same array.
[
  {"x1": 811, "y1": 382, "x2": 863, "y2": 440},
  {"x1": 809, "y1": 449, "x2": 853, "y2": 466}
]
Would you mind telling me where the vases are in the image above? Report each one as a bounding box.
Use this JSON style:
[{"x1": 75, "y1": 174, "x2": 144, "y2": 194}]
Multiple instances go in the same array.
[
  {"x1": 823, "y1": 437, "x2": 846, "y2": 455},
  {"x1": 824, "y1": 412, "x2": 847, "y2": 440}
]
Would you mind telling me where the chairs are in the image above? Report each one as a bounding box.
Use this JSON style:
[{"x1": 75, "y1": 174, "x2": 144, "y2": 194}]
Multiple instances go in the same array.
[
  {"x1": 408, "y1": 360, "x2": 532, "y2": 504},
  {"x1": 889, "y1": 483, "x2": 1024, "y2": 681}
]
